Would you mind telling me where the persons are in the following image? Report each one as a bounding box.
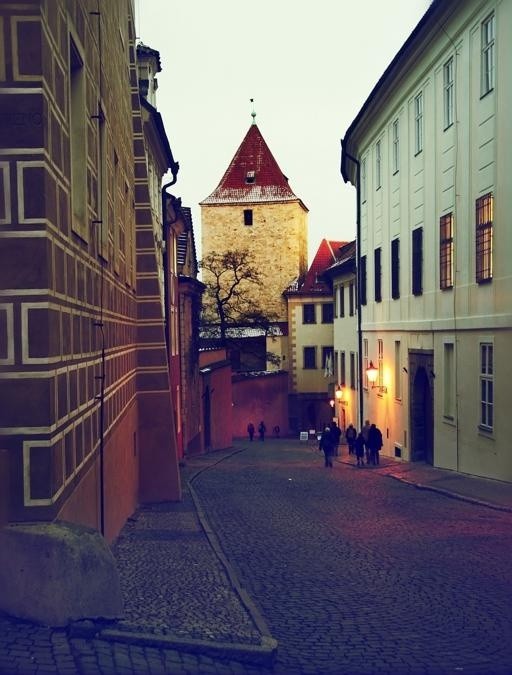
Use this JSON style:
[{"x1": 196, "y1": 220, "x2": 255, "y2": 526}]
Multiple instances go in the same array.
[
  {"x1": 318, "y1": 420, "x2": 382, "y2": 468},
  {"x1": 246, "y1": 420, "x2": 267, "y2": 442}
]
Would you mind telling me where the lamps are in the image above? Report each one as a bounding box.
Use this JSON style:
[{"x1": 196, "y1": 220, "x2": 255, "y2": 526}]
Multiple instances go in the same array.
[
  {"x1": 334, "y1": 386, "x2": 346, "y2": 404},
  {"x1": 363, "y1": 360, "x2": 385, "y2": 392}
]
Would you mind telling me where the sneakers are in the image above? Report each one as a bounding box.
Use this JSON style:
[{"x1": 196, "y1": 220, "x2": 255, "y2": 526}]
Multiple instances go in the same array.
[{"x1": 325, "y1": 463, "x2": 332, "y2": 467}]
